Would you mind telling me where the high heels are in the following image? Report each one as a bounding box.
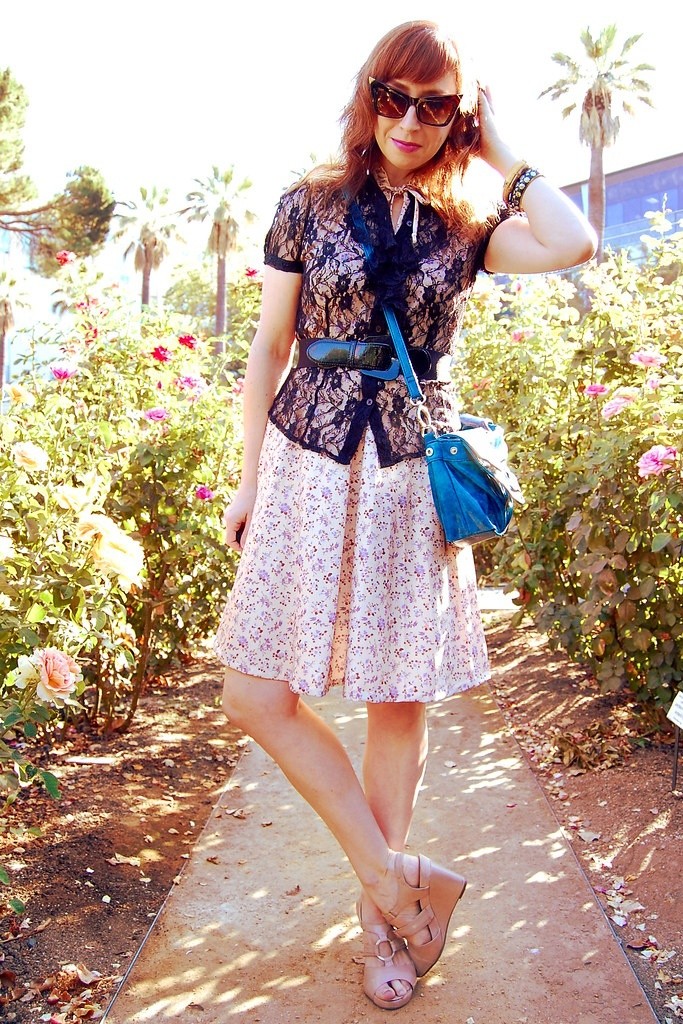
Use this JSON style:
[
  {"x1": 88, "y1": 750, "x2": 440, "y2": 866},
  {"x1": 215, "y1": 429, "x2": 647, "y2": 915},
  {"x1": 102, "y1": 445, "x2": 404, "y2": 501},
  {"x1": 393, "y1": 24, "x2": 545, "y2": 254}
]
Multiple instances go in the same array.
[
  {"x1": 356, "y1": 894, "x2": 416, "y2": 1008},
  {"x1": 381, "y1": 851, "x2": 467, "y2": 978}
]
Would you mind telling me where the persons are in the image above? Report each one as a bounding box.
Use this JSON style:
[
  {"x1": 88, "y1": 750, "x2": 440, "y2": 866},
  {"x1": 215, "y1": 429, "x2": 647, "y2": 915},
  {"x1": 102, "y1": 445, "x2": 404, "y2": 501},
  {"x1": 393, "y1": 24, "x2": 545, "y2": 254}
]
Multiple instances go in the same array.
[{"x1": 207, "y1": 13, "x2": 600, "y2": 1012}]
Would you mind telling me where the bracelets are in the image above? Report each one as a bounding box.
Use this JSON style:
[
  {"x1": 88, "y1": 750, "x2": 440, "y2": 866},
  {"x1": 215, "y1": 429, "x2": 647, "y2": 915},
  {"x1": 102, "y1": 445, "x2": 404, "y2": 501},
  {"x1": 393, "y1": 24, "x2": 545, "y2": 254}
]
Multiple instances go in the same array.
[{"x1": 501, "y1": 159, "x2": 545, "y2": 215}]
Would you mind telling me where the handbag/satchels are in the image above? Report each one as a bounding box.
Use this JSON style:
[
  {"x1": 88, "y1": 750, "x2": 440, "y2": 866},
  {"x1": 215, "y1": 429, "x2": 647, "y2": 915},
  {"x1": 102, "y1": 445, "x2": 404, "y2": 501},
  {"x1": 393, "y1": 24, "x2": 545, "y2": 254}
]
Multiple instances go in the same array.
[{"x1": 424, "y1": 414, "x2": 525, "y2": 542}]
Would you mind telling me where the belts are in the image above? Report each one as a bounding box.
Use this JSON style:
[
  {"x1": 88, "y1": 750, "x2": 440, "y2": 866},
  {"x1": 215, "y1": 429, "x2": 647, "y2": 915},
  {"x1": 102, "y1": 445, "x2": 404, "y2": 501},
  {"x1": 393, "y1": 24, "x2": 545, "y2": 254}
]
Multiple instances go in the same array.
[{"x1": 296, "y1": 338, "x2": 451, "y2": 383}]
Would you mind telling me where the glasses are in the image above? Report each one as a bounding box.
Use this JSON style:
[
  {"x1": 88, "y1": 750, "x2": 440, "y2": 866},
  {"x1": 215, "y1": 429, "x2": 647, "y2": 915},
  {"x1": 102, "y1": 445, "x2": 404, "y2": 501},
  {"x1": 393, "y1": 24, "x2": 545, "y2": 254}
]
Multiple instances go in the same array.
[{"x1": 369, "y1": 76, "x2": 465, "y2": 128}]
